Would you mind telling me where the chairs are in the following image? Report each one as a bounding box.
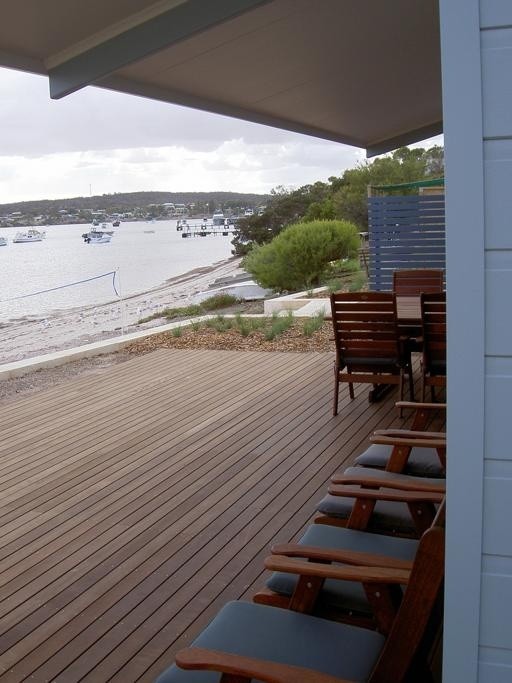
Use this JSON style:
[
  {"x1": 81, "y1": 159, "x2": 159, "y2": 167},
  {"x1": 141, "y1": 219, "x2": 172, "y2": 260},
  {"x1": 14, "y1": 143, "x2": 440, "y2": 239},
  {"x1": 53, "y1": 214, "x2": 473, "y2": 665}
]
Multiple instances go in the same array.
[
  {"x1": 327, "y1": 291, "x2": 414, "y2": 420},
  {"x1": 153, "y1": 495, "x2": 447, "y2": 683},
  {"x1": 354, "y1": 402, "x2": 446, "y2": 478},
  {"x1": 418, "y1": 291, "x2": 446, "y2": 405},
  {"x1": 393, "y1": 271, "x2": 444, "y2": 298},
  {"x1": 252, "y1": 488, "x2": 447, "y2": 632},
  {"x1": 312, "y1": 436, "x2": 446, "y2": 537}
]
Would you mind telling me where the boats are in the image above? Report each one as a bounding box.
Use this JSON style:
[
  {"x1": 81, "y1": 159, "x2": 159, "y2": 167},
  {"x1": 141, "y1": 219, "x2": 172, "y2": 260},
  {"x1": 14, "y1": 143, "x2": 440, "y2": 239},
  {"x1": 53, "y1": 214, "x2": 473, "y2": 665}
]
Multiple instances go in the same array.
[
  {"x1": 92, "y1": 218, "x2": 99, "y2": 226},
  {"x1": 101, "y1": 222, "x2": 106, "y2": 228},
  {"x1": 0, "y1": 236, "x2": 7, "y2": 246},
  {"x1": 113, "y1": 219, "x2": 121, "y2": 227},
  {"x1": 82, "y1": 226, "x2": 115, "y2": 238},
  {"x1": 213, "y1": 209, "x2": 226, "y2": 225},
  {"x1": 83, "y1": 233, "x2": 112, "y2": 244},
  {"x1": 13, "y1": 229, "x2": 46, "y2": 243}
]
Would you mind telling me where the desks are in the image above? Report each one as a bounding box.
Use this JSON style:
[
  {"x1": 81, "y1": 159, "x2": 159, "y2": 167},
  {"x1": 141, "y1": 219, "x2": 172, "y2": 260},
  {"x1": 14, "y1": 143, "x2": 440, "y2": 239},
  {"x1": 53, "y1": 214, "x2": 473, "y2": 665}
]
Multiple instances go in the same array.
[{"x1": 336, "y1": 294, "x2": 446, "y2": 405}]
